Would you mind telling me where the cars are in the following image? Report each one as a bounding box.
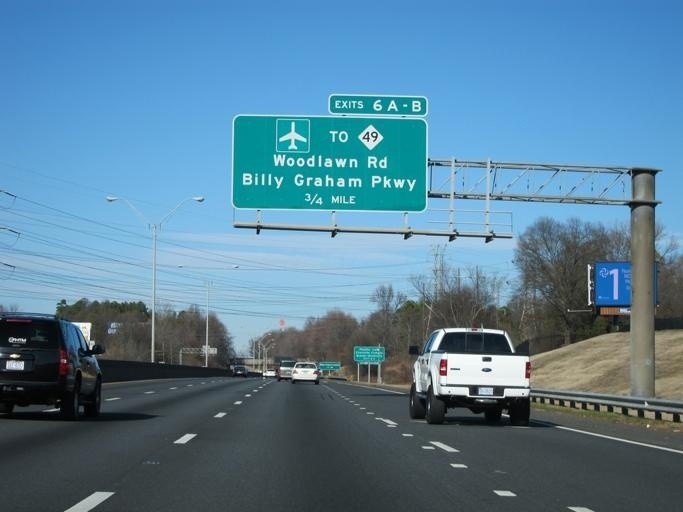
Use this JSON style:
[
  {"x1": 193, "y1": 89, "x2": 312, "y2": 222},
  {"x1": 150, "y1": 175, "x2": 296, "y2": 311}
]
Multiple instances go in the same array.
[
  {"x1": 233, "y1": 366, "x2": 250, "y2": 378},
  {"x1": 291, "y1": 362, "x2": 321, "y2": 386},
  {"x1": 263, "y1": 369, "x2": 278, "y2": 377}
]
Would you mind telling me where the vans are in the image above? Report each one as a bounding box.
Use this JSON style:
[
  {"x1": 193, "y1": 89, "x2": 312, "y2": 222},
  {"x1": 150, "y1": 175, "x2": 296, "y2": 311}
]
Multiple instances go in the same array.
[{"x1": 278, "y1": 360, "x2": 298, "y2": 382}]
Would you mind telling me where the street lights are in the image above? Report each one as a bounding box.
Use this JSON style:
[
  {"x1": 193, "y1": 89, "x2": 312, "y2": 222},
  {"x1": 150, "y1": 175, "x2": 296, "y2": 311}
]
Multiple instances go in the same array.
[
  {"x1": 178, "y1": 264, "x2": 240, "y2": 369},
  {"x1": 105, "y1": 195, "x2": 205, "y2": 363},
  {"x1": 244, "y1": 330, "x2": 276, "y2": 372}
]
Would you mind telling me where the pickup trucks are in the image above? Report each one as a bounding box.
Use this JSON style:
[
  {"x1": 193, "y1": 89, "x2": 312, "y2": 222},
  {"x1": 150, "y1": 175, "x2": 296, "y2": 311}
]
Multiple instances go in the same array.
[{"x1": 408, "y1": 328, "x2": 535, "y2": 427}]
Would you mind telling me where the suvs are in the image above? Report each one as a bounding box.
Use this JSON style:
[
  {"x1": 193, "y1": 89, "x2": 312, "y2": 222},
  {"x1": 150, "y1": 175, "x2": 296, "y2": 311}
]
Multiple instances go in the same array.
[{"x1": 0, "y1": 311, "x2": 106, "y2": 418}]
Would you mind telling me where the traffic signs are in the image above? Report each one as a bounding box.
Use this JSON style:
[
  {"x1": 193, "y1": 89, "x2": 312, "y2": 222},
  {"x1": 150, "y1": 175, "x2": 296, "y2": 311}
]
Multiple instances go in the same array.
[{"x1": 231, "y1": 111, "x2": 427, "y2": 214}]
